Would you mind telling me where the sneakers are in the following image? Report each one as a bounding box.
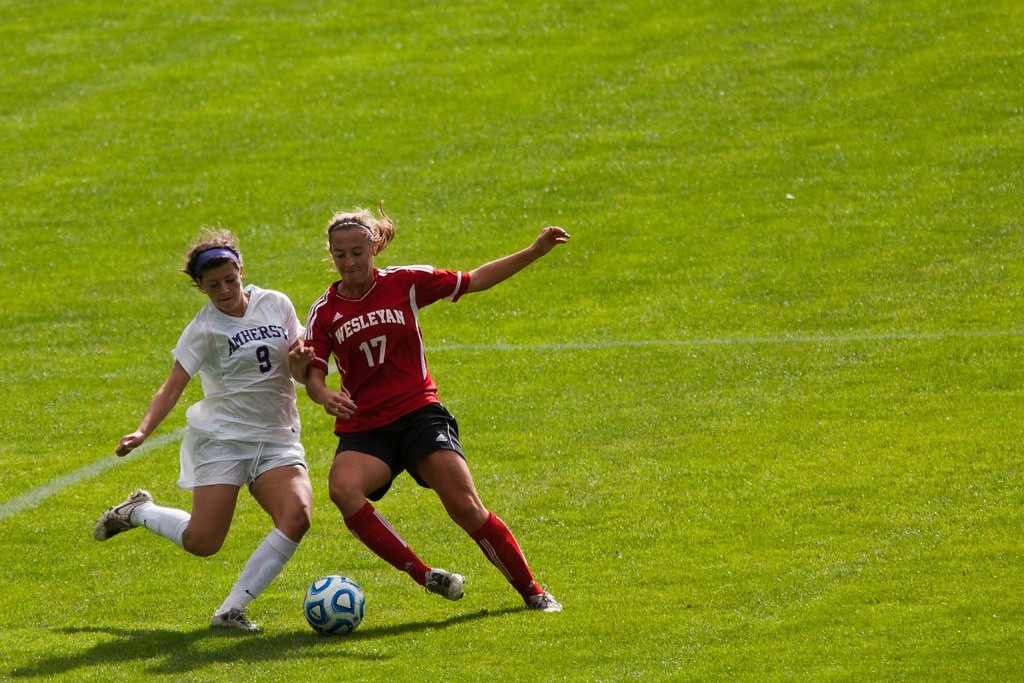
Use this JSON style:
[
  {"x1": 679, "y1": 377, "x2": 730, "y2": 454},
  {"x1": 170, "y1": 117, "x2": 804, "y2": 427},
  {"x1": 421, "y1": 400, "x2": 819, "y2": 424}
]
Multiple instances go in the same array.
[
  {"x1": 530, "y1": 585, "x2": 562, "y2": 613},
  {"x1": 93, "y1": 488, "x2": 152, "y2": 542},
  {"x1": 209, "y1": 607, "x2": 259, "y2": 631},
  {"x1": 425, "y1": 567, "x2": 466, "y2": 601}
]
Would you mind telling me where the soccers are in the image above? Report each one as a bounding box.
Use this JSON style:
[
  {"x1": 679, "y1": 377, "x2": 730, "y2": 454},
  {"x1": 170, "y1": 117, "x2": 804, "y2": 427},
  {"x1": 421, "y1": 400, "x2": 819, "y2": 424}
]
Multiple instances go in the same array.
[{"x1": 303, "y1": 574, "x2": 365, "y2": 637}]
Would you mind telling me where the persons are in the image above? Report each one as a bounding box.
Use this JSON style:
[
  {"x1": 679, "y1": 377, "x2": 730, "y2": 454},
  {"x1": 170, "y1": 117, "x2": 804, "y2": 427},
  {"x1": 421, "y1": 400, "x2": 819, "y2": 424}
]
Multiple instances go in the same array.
[
  {"x1": 305, "y1": 211, "x2": 571, "y2": 613},
  {"x1": 94, "y1": 225, "x2": 316, "y2": 631}
]
